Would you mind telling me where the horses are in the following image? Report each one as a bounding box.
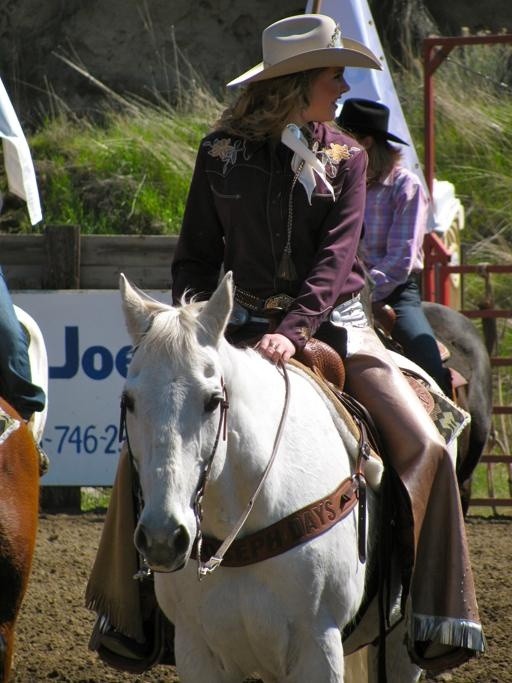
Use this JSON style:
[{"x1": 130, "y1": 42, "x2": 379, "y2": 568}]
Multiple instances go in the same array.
[
  {"x1": 413, "y1": 302, "x2": 492, "y2": 516},
  {"x1": 121, "y1": 271, "x2": 458, "y2": 683}
]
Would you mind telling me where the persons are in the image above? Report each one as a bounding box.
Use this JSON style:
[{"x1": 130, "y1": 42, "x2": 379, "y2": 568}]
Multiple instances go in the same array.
[
  {"x1": 0, "y1": 74, "x2": 50, "y2": 478},
  {"x1": 335, "y1": 96, "x2": 454, "y2": 404},
  {"x1": 86, "y1": 13, "x2": 486, "y2": 670}
]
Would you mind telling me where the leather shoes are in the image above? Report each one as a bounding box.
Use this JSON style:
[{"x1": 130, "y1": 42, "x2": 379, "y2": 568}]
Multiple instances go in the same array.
[
  {"x1": 99, "y1": 626, "x2": 178, "y2": 668},
  {"x1": 405, "y1": 634, "x2": 478, "y2": 674}
]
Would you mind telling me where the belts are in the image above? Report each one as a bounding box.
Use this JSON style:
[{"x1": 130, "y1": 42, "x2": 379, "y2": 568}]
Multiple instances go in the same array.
[{"x1": 234, "y1": 287, "x2": 363, "y2": 318}]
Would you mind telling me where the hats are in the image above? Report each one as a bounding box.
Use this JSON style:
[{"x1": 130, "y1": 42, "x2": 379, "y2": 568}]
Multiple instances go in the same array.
[
  {"x1": 226, "y1": 14, "x2": 383, "y2": 88},
  {"x1": 336, "y1": 97, "x2": 410, "y2": 147}
]
